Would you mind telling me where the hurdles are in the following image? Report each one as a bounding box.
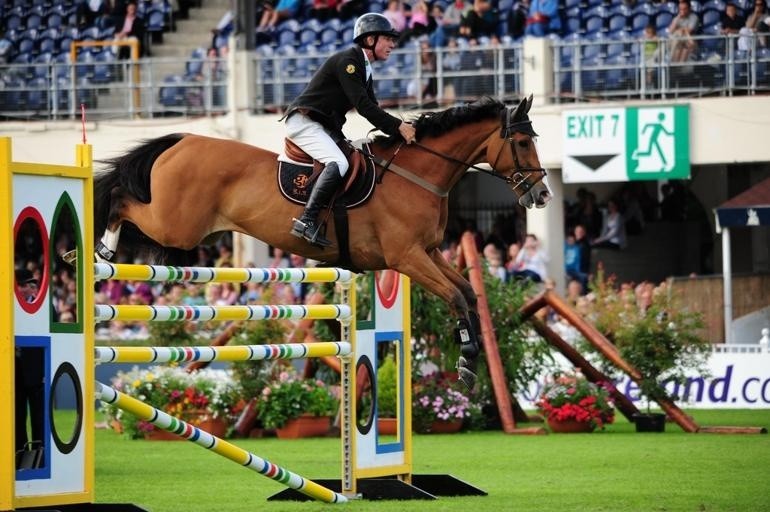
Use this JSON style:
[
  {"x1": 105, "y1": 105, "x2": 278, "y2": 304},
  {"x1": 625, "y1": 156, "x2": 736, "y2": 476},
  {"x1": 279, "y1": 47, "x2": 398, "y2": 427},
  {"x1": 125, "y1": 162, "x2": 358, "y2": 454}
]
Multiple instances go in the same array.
[{"x1": 1, "y1": 137, "x2": 488, "y2": 512}]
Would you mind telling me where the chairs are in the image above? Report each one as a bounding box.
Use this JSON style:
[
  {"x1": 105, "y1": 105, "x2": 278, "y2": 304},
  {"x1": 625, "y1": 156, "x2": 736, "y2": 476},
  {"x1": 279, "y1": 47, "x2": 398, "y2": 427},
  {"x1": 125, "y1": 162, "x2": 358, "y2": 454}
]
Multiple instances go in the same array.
[{"x1": 0, "y1": 0, "x2": 770, "y2": 121}]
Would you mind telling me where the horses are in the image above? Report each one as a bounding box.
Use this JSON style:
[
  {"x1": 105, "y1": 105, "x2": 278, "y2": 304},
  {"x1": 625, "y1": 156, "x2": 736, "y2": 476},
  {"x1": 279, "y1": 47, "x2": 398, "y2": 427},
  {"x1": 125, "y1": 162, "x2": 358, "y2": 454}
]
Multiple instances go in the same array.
[{"x1": 62, "y1": 91, "x2": 553, "y2": 392}]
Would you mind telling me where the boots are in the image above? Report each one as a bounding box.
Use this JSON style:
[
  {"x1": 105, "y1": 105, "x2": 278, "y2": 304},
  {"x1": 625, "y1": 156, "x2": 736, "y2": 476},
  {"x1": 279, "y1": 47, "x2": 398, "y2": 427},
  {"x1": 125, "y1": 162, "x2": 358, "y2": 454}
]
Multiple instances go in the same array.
[{"x1": 289, "y1": 161, "x2": 342, "y2": 247}]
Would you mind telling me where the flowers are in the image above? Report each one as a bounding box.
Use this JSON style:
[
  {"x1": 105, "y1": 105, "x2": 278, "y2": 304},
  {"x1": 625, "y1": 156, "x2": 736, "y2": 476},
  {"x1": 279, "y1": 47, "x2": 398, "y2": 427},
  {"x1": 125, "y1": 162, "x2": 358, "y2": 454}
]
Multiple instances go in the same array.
[
  {"x1": 412, "y1": 385, "x2": 481, "y2": 431},
  {"x1": 254, "y1": 371, "x2": 340, "y2": 432},
  {"x1": 566, "y1": 259, "x2": 712, "y2": 414},
  {"x1": 535, "y1": 368, "x2": 616, "y2": 428},
  {"x1": 104, "y1": 366, "x2": 239, "y2": 439}
]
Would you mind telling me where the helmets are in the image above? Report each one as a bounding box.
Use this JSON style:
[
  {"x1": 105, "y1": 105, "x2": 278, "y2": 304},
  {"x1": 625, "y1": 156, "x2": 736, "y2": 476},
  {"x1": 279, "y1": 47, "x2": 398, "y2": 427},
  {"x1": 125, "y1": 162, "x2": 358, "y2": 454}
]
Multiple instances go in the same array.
[{"x1": 353, "y1": 12, "x2": 400, "y2": 43}]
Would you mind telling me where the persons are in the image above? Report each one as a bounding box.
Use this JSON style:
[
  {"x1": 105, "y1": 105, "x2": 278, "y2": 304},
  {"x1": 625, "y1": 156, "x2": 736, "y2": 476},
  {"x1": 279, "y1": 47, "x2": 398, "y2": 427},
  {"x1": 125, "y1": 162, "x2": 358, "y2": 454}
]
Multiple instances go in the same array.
[
  {"x1": 16, "y1": 268, "x2": 44, "y2": 450},
  {"x1": 638, "y1": 0, "x2": 770, "y2": 85},
  {"x1": 76, "y1": 0, "x2": 143, "y2": 40},
  {"x1": 564, "y1": 180, "x2": 713, "y2": 310},
  {"x1": 278, "y1": 12, "x2": 416, "y2": 246},
  {"x1": 15, "y1": 244, "x2": 341, "y2": 342},
  {"x1": 196, "y1": 0, "x2": 562, "y2": 109},
  {"x1": 483, "y1": 235, "x2": 548, "y2": 283}
]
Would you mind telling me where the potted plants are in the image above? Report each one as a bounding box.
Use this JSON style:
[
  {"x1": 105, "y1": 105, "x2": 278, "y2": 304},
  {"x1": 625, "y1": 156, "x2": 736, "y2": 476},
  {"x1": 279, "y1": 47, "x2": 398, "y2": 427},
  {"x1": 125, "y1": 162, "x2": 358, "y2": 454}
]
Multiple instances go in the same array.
[
  {"x1": 428, "y1": 256, "x2": 555, "y2": 431},
  {"x1": 377, "y1": 353, "x2": 398, "y2": 435}
]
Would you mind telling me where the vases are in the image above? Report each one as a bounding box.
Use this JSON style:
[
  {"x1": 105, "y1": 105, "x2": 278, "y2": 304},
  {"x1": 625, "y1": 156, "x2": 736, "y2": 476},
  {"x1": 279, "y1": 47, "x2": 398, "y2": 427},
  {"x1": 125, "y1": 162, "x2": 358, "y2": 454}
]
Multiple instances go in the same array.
[
  {"x1": 273, "y1": 413, "x2": 333, "y2": 438},
  {"x1": 634, "y1": 413, "x2": 667, "y2": 432},
  {"x1": 547, "y1": 416, "x2": 596, "y2": 433},
  {"x1": 140, "y1": 409, "x2": 228, "y2": 440},
  {"x1": 412, "y1": 416, "x2": 462, "y2": 432}
]
